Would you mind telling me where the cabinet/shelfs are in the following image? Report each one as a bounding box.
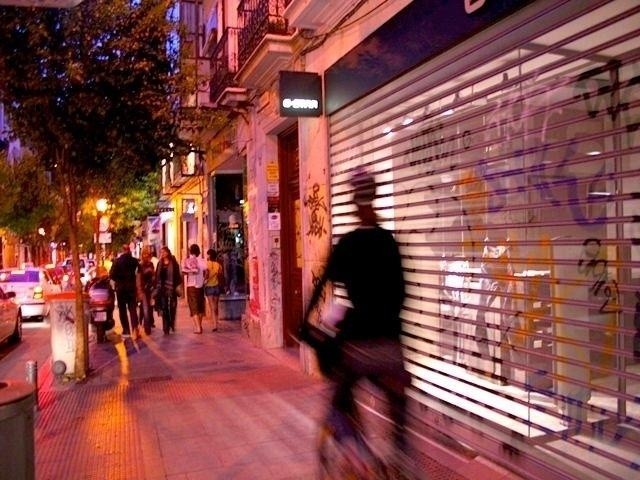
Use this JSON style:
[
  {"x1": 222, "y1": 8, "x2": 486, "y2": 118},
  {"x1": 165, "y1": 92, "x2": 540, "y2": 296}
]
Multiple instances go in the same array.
[{"x1": 506, "y1": 278, "x2": 554, "y2": 393}]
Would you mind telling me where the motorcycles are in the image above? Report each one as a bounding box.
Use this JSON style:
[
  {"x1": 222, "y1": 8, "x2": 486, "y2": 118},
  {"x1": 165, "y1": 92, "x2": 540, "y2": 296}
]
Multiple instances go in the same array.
[{"x1": 84, "y1": 276, "x2": 116, "y2": 344}]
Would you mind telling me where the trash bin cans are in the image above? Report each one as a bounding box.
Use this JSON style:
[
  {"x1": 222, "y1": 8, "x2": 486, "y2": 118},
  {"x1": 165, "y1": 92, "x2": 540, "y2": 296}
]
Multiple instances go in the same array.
[
  {"x1": 44, "y1": 293, "x2": 90, "y2": 376},
  {"x1": 219, "y1": 296, "x2": 250, "y2": 320},
  {"x1": 0, "y1": 380, "x2": 37, "y2": 479}
]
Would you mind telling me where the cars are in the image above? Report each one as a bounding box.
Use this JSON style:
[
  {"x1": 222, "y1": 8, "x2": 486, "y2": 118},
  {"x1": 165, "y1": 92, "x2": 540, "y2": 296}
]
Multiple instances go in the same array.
[{"x1": 0, "y1": 254, "x2": 98, "y2": 348}]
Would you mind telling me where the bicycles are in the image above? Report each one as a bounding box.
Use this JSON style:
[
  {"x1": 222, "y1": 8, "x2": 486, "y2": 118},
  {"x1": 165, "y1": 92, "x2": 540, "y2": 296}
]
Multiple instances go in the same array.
[{"x1": 289, "y1": 325, "x2": 423, "y2": 478}]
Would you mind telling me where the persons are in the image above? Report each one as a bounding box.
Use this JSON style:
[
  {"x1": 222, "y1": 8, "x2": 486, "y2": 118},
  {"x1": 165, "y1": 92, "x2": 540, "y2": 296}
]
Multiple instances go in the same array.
[
  {"x1": 107, "y1": 242, "x2": 222, "y2": 338},
  {"x1": 287, "y1": 172, "x2": 410, "y2": 455}
]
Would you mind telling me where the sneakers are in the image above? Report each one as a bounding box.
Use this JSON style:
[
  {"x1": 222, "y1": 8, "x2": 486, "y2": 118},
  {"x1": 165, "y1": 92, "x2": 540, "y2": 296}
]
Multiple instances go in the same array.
[
  {"x1": 120, "y1": 329, "x2": 131, "y2": 336},
  {"x1": 212, "y1": 328, "x2": 218, "y2": 331},
  {"x1": 194, "y1": 327, "x2": 205, "y2": 334}
]
